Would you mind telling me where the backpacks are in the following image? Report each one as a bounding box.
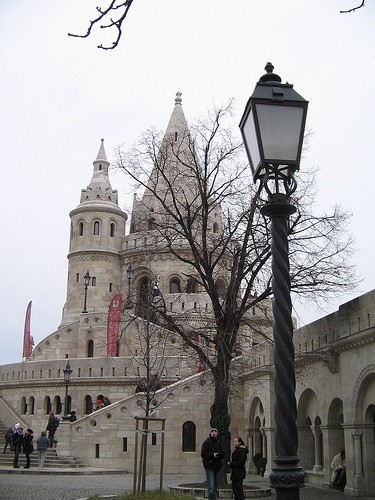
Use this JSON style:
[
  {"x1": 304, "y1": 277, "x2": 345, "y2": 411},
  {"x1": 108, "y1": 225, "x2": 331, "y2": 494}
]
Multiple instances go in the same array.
[{"x1": 54, "y1": 417, "x2": 60, "y2": 427}]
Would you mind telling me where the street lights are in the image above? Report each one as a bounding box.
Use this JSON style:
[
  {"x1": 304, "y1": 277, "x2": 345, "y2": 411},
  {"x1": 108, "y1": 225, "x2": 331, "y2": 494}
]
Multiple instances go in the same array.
[
  {"x1": 124, "y1": 263, "x2": 134, "y2": 310},
  {"x1": 81, "y1": 269, "x2": 91, "y2": 313},
  {"x1": 237, "y1": 61, "x2": 310, "y2": 500},
  {"x1": 62, "y1": 360, "x2": 73, "y2": 421}
]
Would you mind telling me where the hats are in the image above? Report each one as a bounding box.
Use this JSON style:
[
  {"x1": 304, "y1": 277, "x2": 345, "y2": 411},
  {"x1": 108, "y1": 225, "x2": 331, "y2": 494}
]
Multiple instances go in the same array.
[
  {"x1": 27, "y1": 429, "x2": 33, "y2": 434},
  {"x1": 209, "y1": 428, "x2": 218, "y2": 434}
]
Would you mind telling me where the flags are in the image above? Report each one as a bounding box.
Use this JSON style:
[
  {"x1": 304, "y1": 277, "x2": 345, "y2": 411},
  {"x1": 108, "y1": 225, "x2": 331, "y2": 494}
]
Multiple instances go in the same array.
[
  {"x1": 107, "y1": 294, "x2": 122, "y2": 355},
  {"x1": 23, "y1": 301, "x2": 32, "y2": 357}
]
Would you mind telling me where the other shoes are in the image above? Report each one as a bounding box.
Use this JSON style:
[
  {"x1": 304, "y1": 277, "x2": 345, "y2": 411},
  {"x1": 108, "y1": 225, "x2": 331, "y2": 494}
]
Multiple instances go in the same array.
[
  {"x1": 13, "y1": 465, "x2": 20, "y2": 468},
  {"x1": 49, "y1": 445, "x2": 53, "y2": 448},
  {"x1": 24, "y1": 466, "x2": 30, "y2": 468},
  {"x1": 54, "y1": 440, "x2": 57, "y2": 443},
  {"x1": 39, "y1": 465, "x2": 43, "y2": 470}
]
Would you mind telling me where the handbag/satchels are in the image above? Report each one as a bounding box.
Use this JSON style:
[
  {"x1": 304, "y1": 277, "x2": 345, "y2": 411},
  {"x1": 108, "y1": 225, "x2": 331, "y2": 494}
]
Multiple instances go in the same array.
[{"x1": 10, "y1": 446, "x2": 15, "y2": 451}]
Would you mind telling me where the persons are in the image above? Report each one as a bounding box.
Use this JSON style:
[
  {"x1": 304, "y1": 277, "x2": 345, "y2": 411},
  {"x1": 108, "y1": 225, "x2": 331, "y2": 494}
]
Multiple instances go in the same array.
[
  {"x1": 3, "y1": 428, "x2": 14, "y2": 454},
  {"x1": 135, "y1": 377, "x2": 147, "y2": 393},
  {"x1": 22, "y1": 429, "x2": 34, "y2": 468},
  {"x1": 330, "y1": 450, "x2": 346, "y2": 489},
  {"x1": 93, "y1": 400, "x2": 103, "y2": 411},
  {"x1": 198, "y1": 356, "x2": 206, "y2": 372},
  {"x1": 227, "y1": 437, "x2": 249, "y2": 500},
  {"x1": 201, "y1": 427, "x2": 225, "y2": 500},
  {"x1": 253, "y1": 452, "x2": 267, "y2": 476},
  {"x1": 36, "y1": 431, "x2": 51, "y2": 470},
  {"x1": 12, "y1": 427, "x2": 23, "y2": 468},
  {"x1": 45, "y1": 411, "x2": 57, "y2": 448},
  {"x1": 103, "y1": 397, "x2": 111, "y2": 407},
  {"x1": 70, "y1": 411, "x2": 77, "y2": 422},
  {"x1": 150, "y1": 374, "x2": 162, "y2": 391}
]
